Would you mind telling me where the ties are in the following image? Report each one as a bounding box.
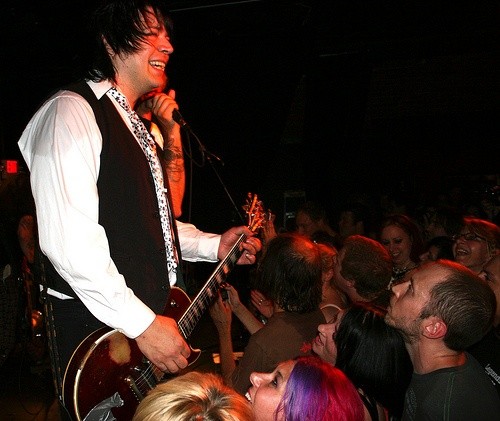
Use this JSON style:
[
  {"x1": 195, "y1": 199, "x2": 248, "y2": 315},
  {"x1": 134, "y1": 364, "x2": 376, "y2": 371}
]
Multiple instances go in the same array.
[{"x1": 108, "y1": 86, "x2": 180, "y2": 275}]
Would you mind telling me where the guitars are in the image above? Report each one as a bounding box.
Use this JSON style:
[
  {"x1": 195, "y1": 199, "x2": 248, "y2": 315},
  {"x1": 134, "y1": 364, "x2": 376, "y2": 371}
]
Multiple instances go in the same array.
[{"x1": 61, "y1": 192, "x2": 266, "y2": 421}]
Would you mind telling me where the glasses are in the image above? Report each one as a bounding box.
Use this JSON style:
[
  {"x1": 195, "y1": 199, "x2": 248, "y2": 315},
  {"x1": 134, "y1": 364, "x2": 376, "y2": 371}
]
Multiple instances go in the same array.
[{"x1": 452, "y1": 232, "x2": 487, "y2": 241}]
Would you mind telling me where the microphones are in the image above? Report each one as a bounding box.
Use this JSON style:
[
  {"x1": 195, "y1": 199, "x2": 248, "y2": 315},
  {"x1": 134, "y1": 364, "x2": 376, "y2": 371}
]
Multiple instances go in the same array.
[{"x1": 172, "y1": 109, "x2": 190, "y2": 131}]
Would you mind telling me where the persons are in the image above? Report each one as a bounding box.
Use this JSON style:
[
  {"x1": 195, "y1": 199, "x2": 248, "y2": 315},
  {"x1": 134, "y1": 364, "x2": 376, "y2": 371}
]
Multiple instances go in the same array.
[
  {"x1": 331, "y1": 234, "x2": 393, "y2": 303},
  {"x1": 375, "y1": 214, "x2": 422, "y2": 268},
  {"x1": 425, "y1": 207, "x2": 458, "y2": 239},
  {"x1": 385, "y1": 258, "x2": 500, "y2": 421},
  {"x1": 208, "y1": 233, "x2": 329, "y2": 397},
  {"x1": 312, "y1": 304, "x2": 414, "y2": 421},
  {"x1": 243, "y1": 355, "x2": 366, "y2": 421},
  {"x1": 132, "y1": 371, "x2": 259, "y2": 421},
  {"x1": 418, "y1": 235, "x2": 456, "y2": 265},
  {"x1": 472, "y1": 254, "x2": 500, "y2": 398},
  {"x1": 15, "y1": 0, "x2": 267, "y2": 421},
  {"x1": 133, "y1": 89, "x2": 186, "y2": 218},
  {"x1": 454, "y1": 216, "x2": 500, "y2": 266},
  {"x1": 294, "y1": 196, "x2": 338, "y2": 238},
  {"x1": 338, "y1": 202, "x2": 373, "y2": 238}
]
